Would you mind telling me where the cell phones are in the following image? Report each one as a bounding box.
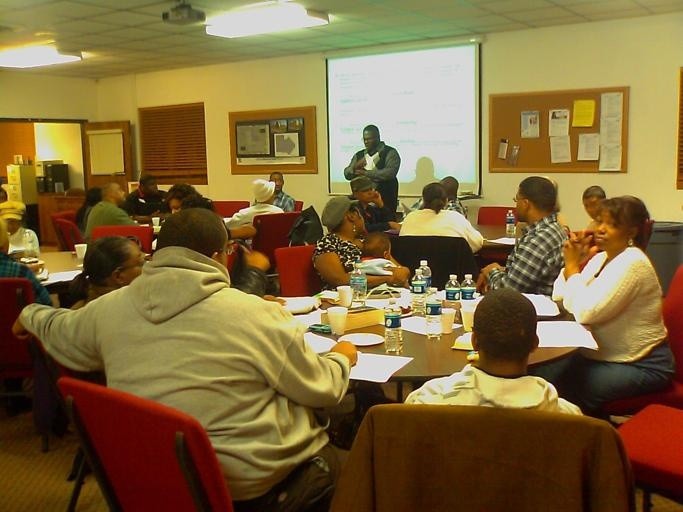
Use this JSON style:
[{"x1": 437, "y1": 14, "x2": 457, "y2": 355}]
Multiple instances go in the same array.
[{"x1": 307, "y1": 323, "x2": 332, "y2": 335}]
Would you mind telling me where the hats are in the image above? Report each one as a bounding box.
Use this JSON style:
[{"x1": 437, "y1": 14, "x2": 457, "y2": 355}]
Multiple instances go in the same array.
[
  {"x1": 350, "y1": 176, "x2": 374, "y2": 192},
  {"x1": 321, "y1": 196, "x2": 359, "y2": 231},
  {"x1": 252, "y1": 179, "x2": 276, "y2": 202},
  {"x1": 0, "y1": 201, "x2": 28, "y2": 220}
]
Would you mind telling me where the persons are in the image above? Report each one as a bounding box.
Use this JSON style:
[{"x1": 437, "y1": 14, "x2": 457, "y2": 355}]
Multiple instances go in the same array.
[
  {"x1": 0, "y1": 200, "x2": 54, "y2": 306},
  {"x1": 64, "y1": 173, "x2": 296, "y2": 309},
  {"x1": 399, "y1": 177, "x2": 483, "y2": 255},
  {"x1": 579, "y1": 184, "x2": 607, "y2": 231},
  {"x1": 10, "y1": 207, "x2": 361, "y2": 510},
  {"x1": 314, "y1": 178, "x2": 410, "y2": 409},
  {"x1": 551, "y1": 194, "x2": 674, "y2": 419},
  {"x1": 402, "y1": 287, "x2": 582, "y2": 416},
  {"x1": 343, "y1": 125, "x2": 400, "y2": 211},
  {"x1": 475, "y1": 176, "x2": 567, "y2": 298}
]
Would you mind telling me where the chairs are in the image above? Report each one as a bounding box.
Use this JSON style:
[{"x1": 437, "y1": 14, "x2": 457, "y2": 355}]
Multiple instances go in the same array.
[
  {"x1": 600, "y1": 265, "x2": 683, "y2": 425},
  {"x1": 0, "y1": 277, "x2": 67, "y2": 453},
  {"x1": 274, "y1": 245, "x2": 323, "y2": 298},
  {"x1": 619, "y1": 401, "x2": 683, "y2": 512},
  {"x1": 49, "y1": 202, "x2": 305, "y2": 273},
  {"x1": 398, "y1": 236, "x2": 476, "y2": 291},
  {"x1": 365, "y1": 404, "x2": 589, "y2": 512},
  {"x1": 474, "y1": 207, "x2": 521, "y2": 268},
  {"x1": 54, "y1": 377, "x2": 234, "y2": 512}
]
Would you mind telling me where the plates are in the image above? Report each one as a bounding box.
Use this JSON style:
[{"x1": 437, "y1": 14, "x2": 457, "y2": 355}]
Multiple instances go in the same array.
[{"x1": 337, "y1": 333, "x2": 385, "y2": 346}]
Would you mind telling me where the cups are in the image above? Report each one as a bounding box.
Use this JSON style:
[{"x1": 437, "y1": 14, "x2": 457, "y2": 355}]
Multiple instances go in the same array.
[
  {"x1": 461, "y1": 307, "x2": 473, "y2": 332},
  {"x1": 151, "y1": 218, "x2": 159, "y2": 232},
  {"x1": 21, "y1": 260, "x2": 44, "y2": 276},
  {"x1": 74, "y1": 244, "x2": 87, "y2": 258},
  {"x1": 336, "y1": 285, "x2": 351, "y2": 307},
  {"x1": 326, "y1": 306, "x2": 347, "y2": 336},
  {"x1": 441, "y1": 307, "x2": 454, "y2": 336}
]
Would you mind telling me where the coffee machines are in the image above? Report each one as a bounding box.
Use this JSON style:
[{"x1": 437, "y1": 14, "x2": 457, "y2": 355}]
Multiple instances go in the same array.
[{"x1": 47, "y1": 164, "x2": 69, "y2": 194}]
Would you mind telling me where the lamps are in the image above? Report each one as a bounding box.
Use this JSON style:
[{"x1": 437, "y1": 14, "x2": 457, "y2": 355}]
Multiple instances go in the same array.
[
  {"x1": 0, "y1": 37, "x2": 81, "y2": 68},
  {"x1": 206, "y1": 1, "x2": 329, "y2": 38}
]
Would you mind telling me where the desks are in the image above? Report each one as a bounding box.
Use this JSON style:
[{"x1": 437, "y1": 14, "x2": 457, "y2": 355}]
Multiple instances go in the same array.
[
  {"x1": 13, "y1": 251, "x2": 90, "y2": 311},
  {"x1": 276, "y1": 283, "x2": 582, "y2": 447},
  {"x1": 386, "y1": 220, "x2": 516, "y2": 266}
]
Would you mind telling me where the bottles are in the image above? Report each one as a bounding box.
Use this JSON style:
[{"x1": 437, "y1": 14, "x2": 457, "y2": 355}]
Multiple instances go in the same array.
[
  {"x1": 446, "y1": 274, "x2": 460, "y2": 321},
  {"x1": 424, "y1": 286, "x2": 440, "y2": 340},
  {"x1": 26, "y1": 240, "x2": 37, "y2": 258},
  {"x1": 461, "y1": 275, "x2": 477, "y2": 305},
  {"x1": 409, "y1": 268, "x2": 425, "y2": 320},
  {"x1": 506, "y1": 210, "x2": 515, "y2": 235},
  {"x1": 350, "y1": 260, "x2": 366, "y2": 308},
  {"x1": 418, "y1": 259, "x2": 430, "y2": 296},
  {"x1": 384, "y1": 298, "x2": 402, "y2": 351}
]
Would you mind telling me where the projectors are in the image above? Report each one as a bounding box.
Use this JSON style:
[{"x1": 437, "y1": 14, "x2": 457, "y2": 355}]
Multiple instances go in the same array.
[{"x1": 161, "y1": 5, "x2": 206, "y2": 26}]
